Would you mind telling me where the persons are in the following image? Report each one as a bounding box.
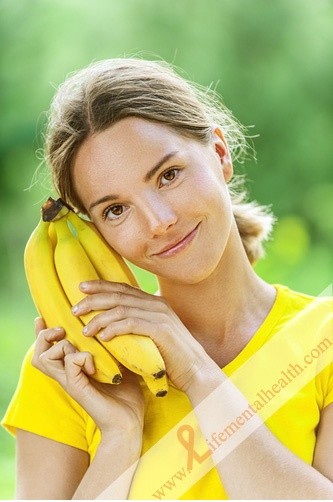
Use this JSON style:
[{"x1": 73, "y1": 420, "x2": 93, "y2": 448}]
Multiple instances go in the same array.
[{"x1": 0, "y1": 47, "x2": 332, "y2": 500}]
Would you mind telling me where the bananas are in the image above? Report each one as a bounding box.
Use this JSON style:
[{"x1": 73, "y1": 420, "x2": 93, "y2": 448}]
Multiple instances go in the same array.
[{"x1": 22, "y1": 198, "x2": 167, "y2": 393}]
